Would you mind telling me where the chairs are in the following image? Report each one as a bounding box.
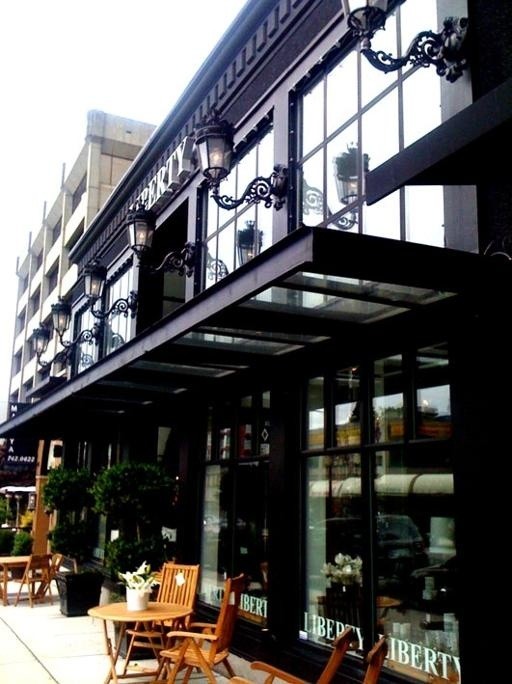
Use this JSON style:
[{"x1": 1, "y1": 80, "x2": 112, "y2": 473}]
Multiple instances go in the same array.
[
  {"x1": 151, "y1": 573, "x2": 244, "y2": 684},
  {"x1": 14, "y1": 554, "x2": 53, "y2": 608},
  {"x1": 28, "y1": 552, "x2": 63, "y2": 603},
  {"x1": 363, "y1": 638, "x2": 387, "y2": 684},
  {"x1": 113, "y1": 562, "x2": 200, "y2": 684},
  {"x1": 229, "y1": 628, "x2": 353, "y2": 684}
]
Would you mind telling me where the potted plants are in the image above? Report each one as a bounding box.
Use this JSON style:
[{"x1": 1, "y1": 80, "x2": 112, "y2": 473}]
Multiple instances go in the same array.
[
  {"x1": 10, "y1": 530, "x2": 33, "y2": 579},
  {"x1": 90, "y1": 460, "x2": 180, "y2": 660},
  {"x1": 41, "y1": 463, "x2": 104, "y2": 616}
]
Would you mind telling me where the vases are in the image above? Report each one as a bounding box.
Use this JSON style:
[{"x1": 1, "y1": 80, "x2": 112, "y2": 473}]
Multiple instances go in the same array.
[{"x1": 126, "y1": 590, "x2": 148, "y2": 611}]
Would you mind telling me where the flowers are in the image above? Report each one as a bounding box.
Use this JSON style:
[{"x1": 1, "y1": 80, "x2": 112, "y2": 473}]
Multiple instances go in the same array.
[
  {"x1": 320, "y1": 552, "x2": 363, "y2": 593},
  {"x1": 116, "y1": 560, "x2": 160, "y2": 593}
]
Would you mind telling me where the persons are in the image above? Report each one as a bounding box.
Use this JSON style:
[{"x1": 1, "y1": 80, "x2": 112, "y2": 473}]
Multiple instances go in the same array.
[
  {"x1": 418, "y1": 527, "x2": 459, "y2": 631},
  {"x1": 375, "y1": 485, "x2": 430, "y2": 617}
]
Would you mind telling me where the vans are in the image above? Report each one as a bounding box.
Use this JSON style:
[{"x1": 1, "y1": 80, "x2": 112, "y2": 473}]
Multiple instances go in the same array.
[{"x1": 309, "y1": 514, "x2": 431, "y2": 580}]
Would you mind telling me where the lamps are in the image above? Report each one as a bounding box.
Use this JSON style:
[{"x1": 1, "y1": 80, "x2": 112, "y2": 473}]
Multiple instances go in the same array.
[
  {"x1": 33, "y1": 321, "x2": 72, "y2": 368},
  {"x1": 81, "y1": 255, "x2": 135, "y2": 318},
  {"x1": 204, "y1": 217, "x2": 264, "y2": 280},
  {"x1": 51, "y1": 294, "x2": 98, "y2": 346},
  {"x1": 302, "y1": 142, "x2": 369, "y2": 229},
  {"x1": 194, "y1": 108, "x2": 288, "y2": 212},
  {"x1": 340, "y1": 0, "x2": 470, "y2": 83},
  {"x1": 126, "y1": 203, "x2": 194, "y2": 277}
]
